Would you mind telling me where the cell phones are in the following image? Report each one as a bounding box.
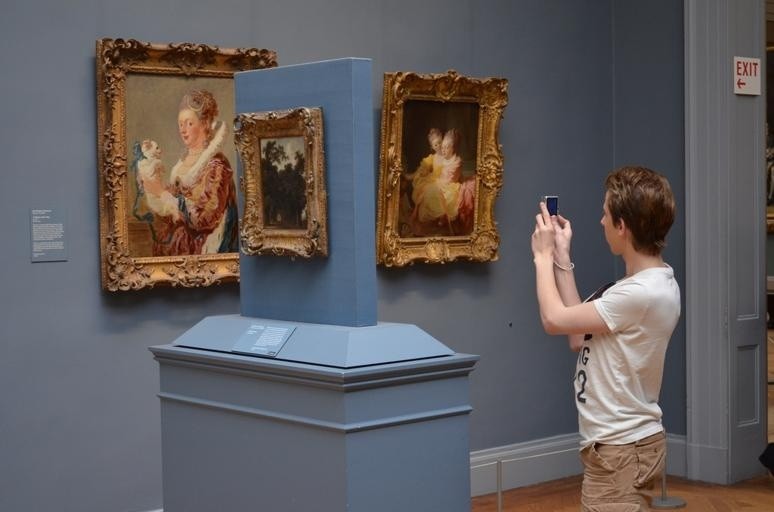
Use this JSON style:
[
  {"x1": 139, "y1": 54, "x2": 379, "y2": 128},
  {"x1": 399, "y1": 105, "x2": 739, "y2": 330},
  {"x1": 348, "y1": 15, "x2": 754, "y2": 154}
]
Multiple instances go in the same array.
[{"x1": 544, "y1": 195, "x2": 559, "y2": 216}]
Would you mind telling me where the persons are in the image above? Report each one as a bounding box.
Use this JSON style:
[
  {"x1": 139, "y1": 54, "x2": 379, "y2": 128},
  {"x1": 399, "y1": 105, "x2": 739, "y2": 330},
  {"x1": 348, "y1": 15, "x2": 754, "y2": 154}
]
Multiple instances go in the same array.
[
  {"x1": 413, "y1": 128, "x2": 474, "y2": 235},
  {"x1": 137, "y1": 90, "x2": 238, "y2": 256},
  {"x1": 531, "y1": 166, "x2": 681, "y2": 512}
]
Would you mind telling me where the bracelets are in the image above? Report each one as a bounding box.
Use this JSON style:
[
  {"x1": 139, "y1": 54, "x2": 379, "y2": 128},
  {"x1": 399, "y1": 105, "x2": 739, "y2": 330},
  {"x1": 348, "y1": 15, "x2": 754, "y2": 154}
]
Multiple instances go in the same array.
[{"x1": 553, "y1": 260, "x2": 574, "y2": 271}]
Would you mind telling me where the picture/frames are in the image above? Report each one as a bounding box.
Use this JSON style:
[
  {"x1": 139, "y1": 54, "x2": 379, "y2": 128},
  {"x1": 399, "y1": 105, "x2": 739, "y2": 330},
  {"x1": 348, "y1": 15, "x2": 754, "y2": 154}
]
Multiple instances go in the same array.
[
  {"x1": 374, "y1": 67, "x2": 508, "y2": 268},
  {"x1": 95, "y1": 37, "x2": 280, "y2": 292},
  {"x1": 232, "y1": 106, "x2": 327, "y2": 261}
]
